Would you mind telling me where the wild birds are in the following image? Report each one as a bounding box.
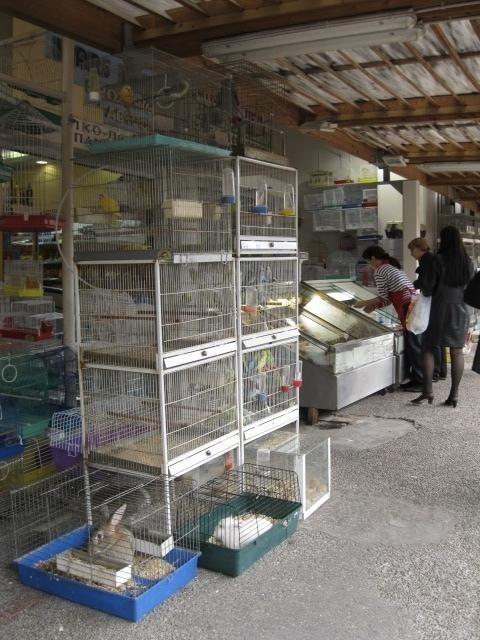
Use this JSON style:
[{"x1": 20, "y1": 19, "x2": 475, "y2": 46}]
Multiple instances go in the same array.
[{"x1": 97, "y1": 192, "x2": 122, "y2": 224}]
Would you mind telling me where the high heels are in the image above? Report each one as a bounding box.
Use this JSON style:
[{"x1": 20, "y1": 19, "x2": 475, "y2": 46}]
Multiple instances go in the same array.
[
  {"x1": 410, "y1": 392, "x2": 435, "y2": 404},
  {"x1": 445, "y1": 392, "x2": 458, "y2": 408}
]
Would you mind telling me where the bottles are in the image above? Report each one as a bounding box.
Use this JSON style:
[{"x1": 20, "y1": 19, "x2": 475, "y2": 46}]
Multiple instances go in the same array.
[
  {"x1": 222, "y1": 168, "x2": 294, "y2": 214},
  {"x1": 257, "y1": 360, "x2": 303, "y2": 395},
  {"x1": 257, "y1": 449, "x2": 270, "y2": 474}
]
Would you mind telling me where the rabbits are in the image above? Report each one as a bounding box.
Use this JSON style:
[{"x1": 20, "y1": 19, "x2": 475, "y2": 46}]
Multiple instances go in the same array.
[
  {"x1": 214, "y1": 514, "x2": 274, "y2": 551},
  {"x1": 87, "y1": 503, "x2": 136, "y2": 566}
]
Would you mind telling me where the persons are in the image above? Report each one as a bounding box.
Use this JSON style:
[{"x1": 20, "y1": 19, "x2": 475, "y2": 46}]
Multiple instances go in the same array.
[
  {"x1": 408, "y1": 238, "x2": 447, "y2": 381},
  {"x1": 353, "y1": 246, "x2": 425, "y2": 391},
  {"x1": 464, "y1": 271, "x2": 480, "y2": 373},
  {"x1": 411, "y1": 226, "x2": 473, "y2": 408}
]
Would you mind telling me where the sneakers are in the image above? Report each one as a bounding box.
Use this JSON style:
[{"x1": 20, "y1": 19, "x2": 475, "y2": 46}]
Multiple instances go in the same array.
[{"x1": 432, "y1": 373, "x2": 448, "y2": 382}]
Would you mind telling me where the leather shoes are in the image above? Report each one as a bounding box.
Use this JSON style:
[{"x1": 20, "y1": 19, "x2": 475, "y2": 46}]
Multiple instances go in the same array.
[{"x1": 399, "y1": 380, "x2": 425, "y2": 392}]
